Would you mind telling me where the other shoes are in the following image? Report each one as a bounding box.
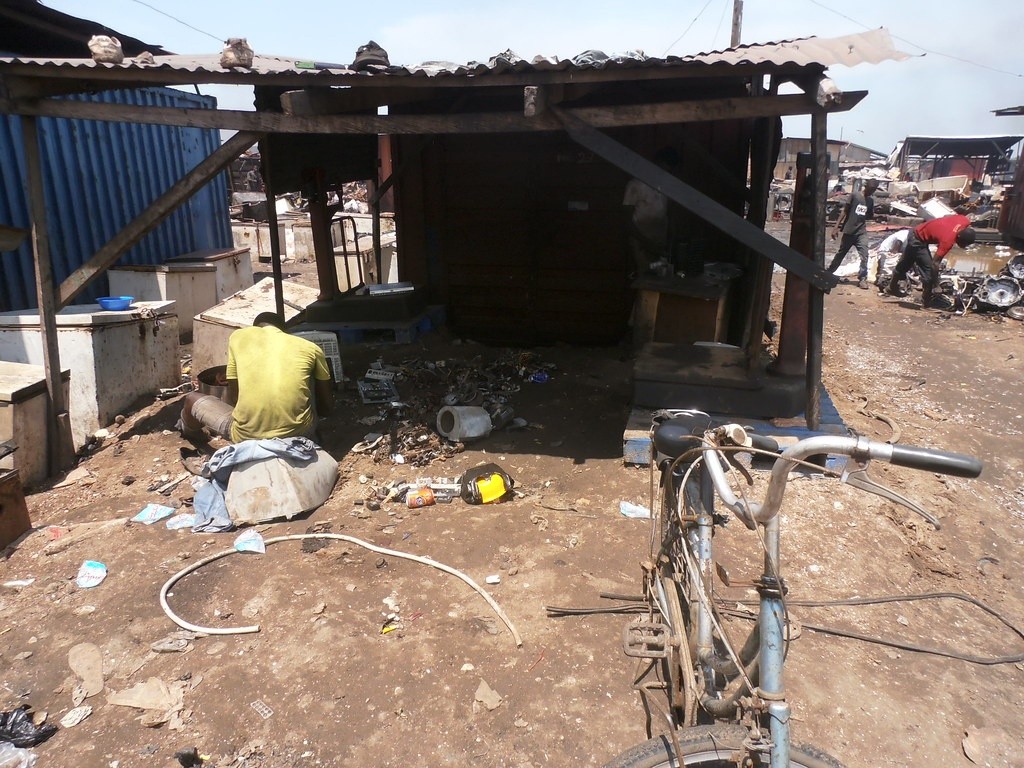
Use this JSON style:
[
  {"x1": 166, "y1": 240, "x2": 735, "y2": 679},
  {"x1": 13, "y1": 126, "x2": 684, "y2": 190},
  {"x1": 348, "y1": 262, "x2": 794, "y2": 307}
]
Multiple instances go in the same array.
[
  {"x1": 858, "y1": 279, "x2": 871, "y2": 289},
  {"x1": 888, "y1": 285, "x2": 951, "y2": 307},
  {"x1": 180, "y1": 446, "x2": 216, "y2": 459}
]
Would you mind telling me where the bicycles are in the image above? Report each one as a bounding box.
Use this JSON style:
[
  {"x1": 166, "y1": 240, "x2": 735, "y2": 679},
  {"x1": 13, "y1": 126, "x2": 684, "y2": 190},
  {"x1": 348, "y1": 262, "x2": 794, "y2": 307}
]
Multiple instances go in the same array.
[{"x1": 595, "y1": 408, "x2": 984, "y2": 767}]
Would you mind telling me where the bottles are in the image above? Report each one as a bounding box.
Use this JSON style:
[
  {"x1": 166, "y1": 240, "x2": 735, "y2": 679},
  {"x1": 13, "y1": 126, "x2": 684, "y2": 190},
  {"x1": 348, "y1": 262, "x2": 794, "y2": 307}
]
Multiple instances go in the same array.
[{"x1": 433, "y1": 489, "x2": 453, "y2": 503}]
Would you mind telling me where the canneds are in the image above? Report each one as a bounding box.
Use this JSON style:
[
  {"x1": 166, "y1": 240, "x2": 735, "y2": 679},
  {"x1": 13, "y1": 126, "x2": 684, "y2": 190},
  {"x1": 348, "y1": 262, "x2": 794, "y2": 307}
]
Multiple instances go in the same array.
[
  {"x1": 406, "y1": 489, "x2": 435, "y2": 508},
  {"x1": 529, "y1": 372, "x2": 548, "y2": 382}
]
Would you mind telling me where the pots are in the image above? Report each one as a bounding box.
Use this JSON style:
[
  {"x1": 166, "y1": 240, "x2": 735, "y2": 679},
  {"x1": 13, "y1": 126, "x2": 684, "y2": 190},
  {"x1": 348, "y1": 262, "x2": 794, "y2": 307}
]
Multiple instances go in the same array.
[{"x1": 197, "y1": 364, "x2": 231, "y2": 407}]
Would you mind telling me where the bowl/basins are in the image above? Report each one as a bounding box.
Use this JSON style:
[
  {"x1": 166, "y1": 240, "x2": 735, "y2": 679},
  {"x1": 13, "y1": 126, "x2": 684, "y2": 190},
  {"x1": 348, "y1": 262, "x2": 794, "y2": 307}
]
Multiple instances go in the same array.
[{"x1": 96, "y1": 295, "x2": 135, "y2": 312}]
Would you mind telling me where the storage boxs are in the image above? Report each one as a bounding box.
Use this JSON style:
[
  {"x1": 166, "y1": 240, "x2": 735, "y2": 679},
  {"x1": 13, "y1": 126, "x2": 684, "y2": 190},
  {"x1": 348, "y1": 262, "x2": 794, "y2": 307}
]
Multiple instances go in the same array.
[
  {"x1": 0, "y1": 189, "x2": 423, "y2": 557},
  {"x1": 888, "y1": 174, "x2": 968, "y2": 222}
]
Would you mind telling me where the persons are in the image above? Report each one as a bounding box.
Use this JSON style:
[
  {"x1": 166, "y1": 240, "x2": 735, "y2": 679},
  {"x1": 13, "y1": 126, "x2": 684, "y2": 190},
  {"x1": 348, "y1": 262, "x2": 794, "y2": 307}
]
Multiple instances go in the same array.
[
  {"x1": 180, "y1": 312, "x2": 339, "y2": 477},
  {"x1": 904, "y1": 172, "x2": 912, "y2": 183},
  {"x1": 825, "y1": 177, "x2": 880, "y2": 290},
  {"x1": 883, "y1": 212, "x2": 976, "y2": 308},
  {"x1": 784, "y1": 166, "x2": 792, "y2": 181}
]
